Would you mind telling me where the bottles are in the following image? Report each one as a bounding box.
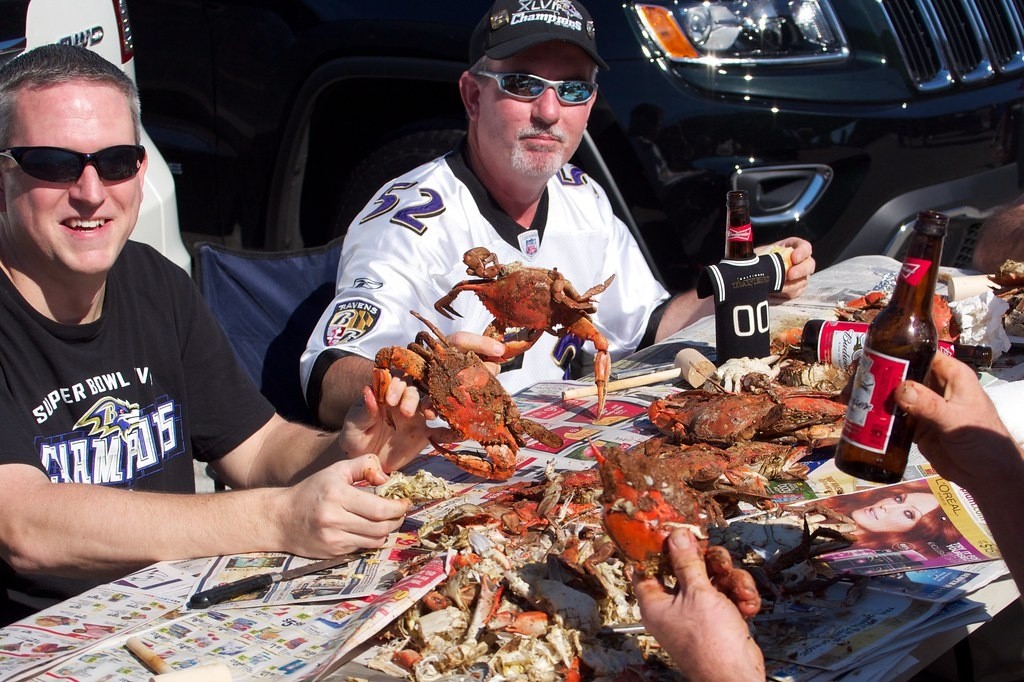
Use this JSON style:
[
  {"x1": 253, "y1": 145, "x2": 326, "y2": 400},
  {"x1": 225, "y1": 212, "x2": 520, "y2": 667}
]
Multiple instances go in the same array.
[
  {"x1": 800, "y1": 320, "x2": 992, "y2": 374},
  {"x1": 835, "y1": 208, "x2": 951, "y2": 485},
  {"x1": 714, "y1": 190, "x2": 770, "y2": 369}
]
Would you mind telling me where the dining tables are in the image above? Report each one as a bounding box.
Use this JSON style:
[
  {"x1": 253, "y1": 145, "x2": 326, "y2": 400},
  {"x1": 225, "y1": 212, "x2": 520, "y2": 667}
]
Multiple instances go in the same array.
[{"x1": 0, "y1": 250, "x2": 1024, "y2": 681}]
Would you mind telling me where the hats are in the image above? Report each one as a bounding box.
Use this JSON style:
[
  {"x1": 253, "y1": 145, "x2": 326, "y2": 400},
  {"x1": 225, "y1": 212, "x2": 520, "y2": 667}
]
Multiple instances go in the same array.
[{"x1": 472, "y1": 2, "x2": 605, "y2": 69}]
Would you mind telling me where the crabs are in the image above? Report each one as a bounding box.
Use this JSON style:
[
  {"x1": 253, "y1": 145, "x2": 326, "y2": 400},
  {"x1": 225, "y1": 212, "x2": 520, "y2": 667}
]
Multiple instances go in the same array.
[
  {"x1": 587, "y1": 328, "x2": 851, "y2": 589},
  {"x1": 370, "y1": 245, "x2": 615, "y2": 484}
]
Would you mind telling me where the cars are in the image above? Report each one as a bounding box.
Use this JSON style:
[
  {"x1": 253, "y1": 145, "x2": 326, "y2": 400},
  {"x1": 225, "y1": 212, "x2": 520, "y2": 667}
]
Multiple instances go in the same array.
[{"x1": 598, "y1": 1, "x2": 1024, "y2": 232}]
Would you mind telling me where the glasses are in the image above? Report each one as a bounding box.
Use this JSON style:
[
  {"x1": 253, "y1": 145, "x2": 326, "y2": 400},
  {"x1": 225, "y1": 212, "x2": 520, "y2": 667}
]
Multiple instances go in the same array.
[
  {"x1": 475, "y1": 73, "x2": 598, "y2": 103},
  {"x1": 1, "y1": 148, "x2": 147, "y2": 184}
]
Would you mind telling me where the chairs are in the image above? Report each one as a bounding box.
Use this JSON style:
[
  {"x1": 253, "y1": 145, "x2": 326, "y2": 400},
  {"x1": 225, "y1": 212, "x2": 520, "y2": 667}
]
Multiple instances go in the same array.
[{"x1": 194, "y1": 236, "x2": 343, "y2": 493}]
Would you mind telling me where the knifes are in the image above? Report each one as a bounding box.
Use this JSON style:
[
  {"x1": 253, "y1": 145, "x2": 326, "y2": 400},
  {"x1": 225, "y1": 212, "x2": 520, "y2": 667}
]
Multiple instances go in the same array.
[{"x1": 190, "y1": 552, "x2": 365, "y2": 609}]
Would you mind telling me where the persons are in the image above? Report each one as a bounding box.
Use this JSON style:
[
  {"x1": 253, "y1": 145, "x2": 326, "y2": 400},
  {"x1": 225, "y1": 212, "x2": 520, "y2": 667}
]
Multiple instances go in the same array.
[
  {"x1": 299, "y1": 0, "x2": 816, "y2": 429},
  {"x1": 0, "y1": 43, "x2": 469, "y2": 627},
  {"x1": 633, "y1": 351, "x2": 1024, "y2": 682},
  {"x1": 809, "y1": 482, "x2": 961, "y2": 557}
]
